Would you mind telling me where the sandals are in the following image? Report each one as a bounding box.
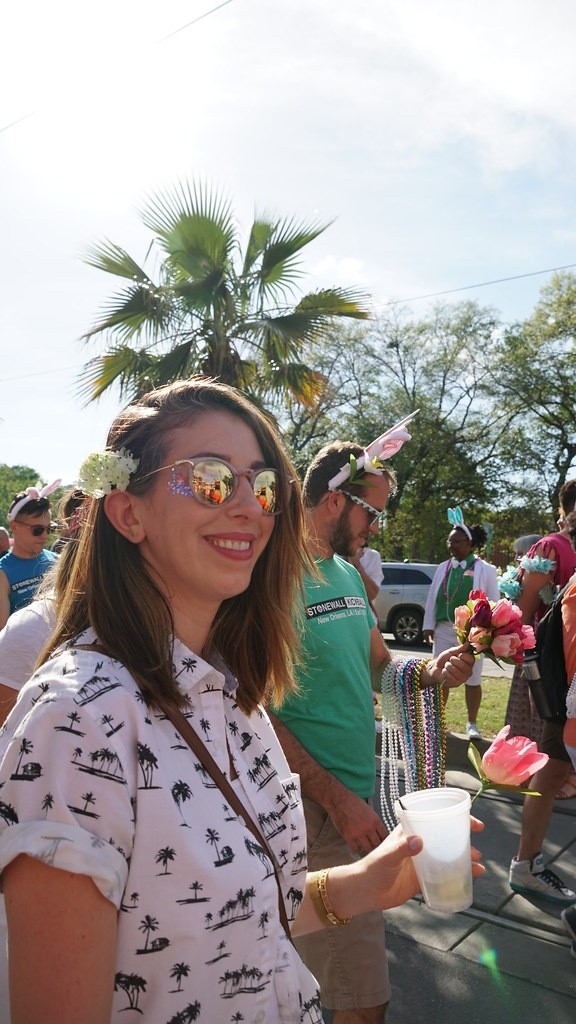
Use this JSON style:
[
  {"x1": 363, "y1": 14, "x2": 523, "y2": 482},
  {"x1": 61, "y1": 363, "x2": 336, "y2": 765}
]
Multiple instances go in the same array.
[{"x1": 554, "y1": 775, "x2": 576, "y2": 800}]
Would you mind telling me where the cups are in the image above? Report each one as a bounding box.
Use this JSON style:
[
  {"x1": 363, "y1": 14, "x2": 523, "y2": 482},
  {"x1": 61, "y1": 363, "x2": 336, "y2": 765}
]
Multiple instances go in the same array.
[{"x1": 394, "y1": 788, "x2": 473, "y2": 914}]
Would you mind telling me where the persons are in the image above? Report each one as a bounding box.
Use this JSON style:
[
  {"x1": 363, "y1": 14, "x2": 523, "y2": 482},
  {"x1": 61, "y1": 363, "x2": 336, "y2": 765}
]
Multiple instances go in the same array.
[
  {"x1": 262, "y1": 445, "x2": 476, "y2": 1024},
  {"x1": 0, "y1": 379, "x2": 484, "y2": 1024},
  {"x1": 338, "y1": 539, "x2": 383, "y2": 626},
  {"x1": 0, "y1": 481, "x2": 94, "y2": 728},
  {"x1": 424, "y1": 480, "x2": 576, "y2": 947}
]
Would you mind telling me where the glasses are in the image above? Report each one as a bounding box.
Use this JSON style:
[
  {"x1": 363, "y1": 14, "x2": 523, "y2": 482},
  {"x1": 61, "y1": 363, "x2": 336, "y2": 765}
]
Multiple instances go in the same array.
[
  {"x1": 330, "y1": 486, "x2": 387, "y2": 528},
  {"x1": 15, "y1": 519, "x2": 58, "y2": 537},
  {"x1": 124, "y1": 456, "x2": 296, "y2": 516}
]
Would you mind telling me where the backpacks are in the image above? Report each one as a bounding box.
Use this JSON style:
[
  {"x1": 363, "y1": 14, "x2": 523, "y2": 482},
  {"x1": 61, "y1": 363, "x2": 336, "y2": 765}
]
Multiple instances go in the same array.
[{"x1": 521, "y1": 580, "x2": 572, "y2": 725}]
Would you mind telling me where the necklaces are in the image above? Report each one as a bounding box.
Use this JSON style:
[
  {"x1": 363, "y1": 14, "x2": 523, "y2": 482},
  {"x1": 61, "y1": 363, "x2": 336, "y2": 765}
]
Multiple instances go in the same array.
[
  {"x1": 442, "y1": 559, "x2": 480, "y2": 604},
  {"x1": 381, "y1": 655, "x2": 448, "y2": 833}
]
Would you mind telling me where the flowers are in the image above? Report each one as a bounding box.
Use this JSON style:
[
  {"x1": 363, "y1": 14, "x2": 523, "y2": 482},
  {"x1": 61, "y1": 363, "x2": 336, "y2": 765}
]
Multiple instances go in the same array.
[
  {"x1": 467, "y1": 722, "x2": 548, "y2": 803},
  {"x1": 76, "y1": 446, "x2": 140, "y2": 500},
  {"x1": 310, "y1": 448, "x2": 387, "y2": 513},
  {"x1": 496, "y1": 565, "x2": 524, "y2": 600},
  {"x1": 538, "y1": 585, "x2": 560, "y2": 606},
  {"x1": 521, "y1": 556, "x2": 557, "y2": 574},
  {"x1": 441, "y1": 587, "x2": 536, "y2": 690}
]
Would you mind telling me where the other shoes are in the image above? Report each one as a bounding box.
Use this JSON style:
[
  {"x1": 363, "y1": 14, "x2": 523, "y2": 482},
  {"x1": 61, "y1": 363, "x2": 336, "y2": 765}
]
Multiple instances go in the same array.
[
  {"x1": 561, "y1": 906, "x2": 576, "y2": 941},
  {"x1": 571, "y1": 944, "x2": 576, "y2": 959}
]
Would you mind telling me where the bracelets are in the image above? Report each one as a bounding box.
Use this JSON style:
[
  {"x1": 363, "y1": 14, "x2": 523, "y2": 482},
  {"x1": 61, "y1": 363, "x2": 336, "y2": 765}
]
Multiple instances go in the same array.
[{"x1": 309, "y1": 866, "x2": 351, "y2": 928}]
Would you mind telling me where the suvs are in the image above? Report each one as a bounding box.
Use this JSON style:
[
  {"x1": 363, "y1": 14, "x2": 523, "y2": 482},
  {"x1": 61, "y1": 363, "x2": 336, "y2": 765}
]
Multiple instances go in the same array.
[{"x1": 372, "y1": 559, "x2": 440, "y2": 647}]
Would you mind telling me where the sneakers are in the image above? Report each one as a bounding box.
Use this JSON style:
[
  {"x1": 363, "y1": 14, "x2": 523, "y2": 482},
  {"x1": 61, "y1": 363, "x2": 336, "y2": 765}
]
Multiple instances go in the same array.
[
  {"x1": 508, "y1": 852, "x2": 576, "y2": 906},
  {"x1": 466, "y1": 723, "x2": 481, "y2": 740}
]
